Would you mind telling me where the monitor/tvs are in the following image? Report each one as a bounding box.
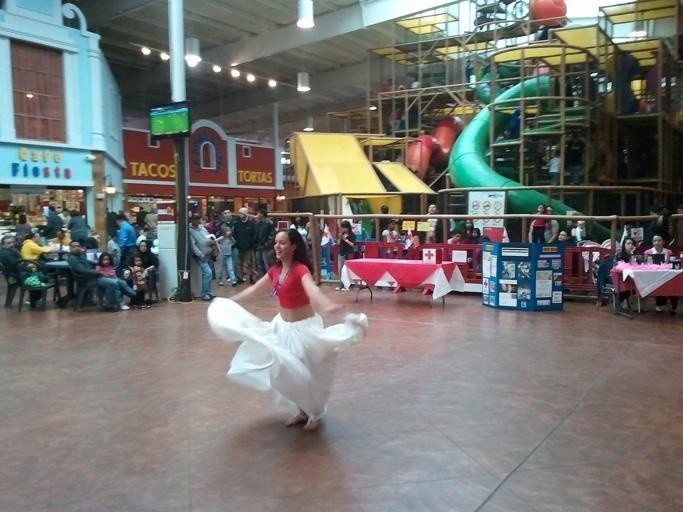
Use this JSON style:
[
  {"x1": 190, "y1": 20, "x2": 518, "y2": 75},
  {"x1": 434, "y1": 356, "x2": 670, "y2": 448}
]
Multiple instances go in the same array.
[{"x1": 148, "y1": 100, "x2": 191, "y2": 140}]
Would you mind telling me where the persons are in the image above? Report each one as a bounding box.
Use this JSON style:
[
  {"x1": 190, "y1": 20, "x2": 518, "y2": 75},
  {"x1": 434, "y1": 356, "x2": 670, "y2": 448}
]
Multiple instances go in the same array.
[
  {"x1": 207, "y1": 228, "x2": 368, "y2": 433},
  {"x1": 0, "y1": 203, "x2": 683, "y2": 311},
  {"x1": 540, "y1": 150, "x2": 561, "y2": 185},
  {"x1": 567, "y1": 133, "x2": 585, "y2": 185}
]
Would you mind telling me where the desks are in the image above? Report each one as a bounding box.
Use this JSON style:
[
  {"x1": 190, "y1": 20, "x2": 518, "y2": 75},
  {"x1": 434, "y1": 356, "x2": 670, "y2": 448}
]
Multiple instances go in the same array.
[
  {"x1": 341, "y1": 258, "x2": 466, "y2": 310},
  {"x1": 612, "y1": 269, "x2": 683, "y2": 314}
]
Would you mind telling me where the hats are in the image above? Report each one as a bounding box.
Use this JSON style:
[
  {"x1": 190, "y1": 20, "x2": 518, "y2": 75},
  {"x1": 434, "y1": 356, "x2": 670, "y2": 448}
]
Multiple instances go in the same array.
[{"x1": 239, "y1": 207, "x2": 249, "y2": 216}]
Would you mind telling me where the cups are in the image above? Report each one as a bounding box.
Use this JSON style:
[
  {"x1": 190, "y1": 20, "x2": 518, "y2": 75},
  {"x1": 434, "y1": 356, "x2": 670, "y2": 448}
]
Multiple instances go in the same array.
[
  {"x1": 86, "y1": 253, "x2": 95, "y2": 261},
  {"x1": 97, "y1": 253, "x2": 101, "y2": 259},
  {"x1": 669, "y1": 256, "x2": 676, "y2": 262}
]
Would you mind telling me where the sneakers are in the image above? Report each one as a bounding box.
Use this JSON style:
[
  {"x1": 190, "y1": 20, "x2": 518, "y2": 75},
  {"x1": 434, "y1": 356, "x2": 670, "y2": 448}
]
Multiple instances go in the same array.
[
  {"x1": 340, "y1": 287, "x2": 351, "y2": 291},
  {"x1": 285, "y1": 414, "x2": 308, "y2": 427},
  {"x1": 335, "y1": 285, "x2": 343, "y2": 291},
  {"x1": 304, "y1": 417, "x2": 321, "y2": 431},
  {"x1": 199, "y1": 290, "x2": 216, "y2": 301},
  {"x1": 106, "y1": 291, "x2": 152, "y2": 312}
]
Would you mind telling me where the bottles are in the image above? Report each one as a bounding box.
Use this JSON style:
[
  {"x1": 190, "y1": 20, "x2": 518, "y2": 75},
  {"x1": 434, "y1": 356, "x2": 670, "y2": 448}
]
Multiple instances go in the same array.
[
  {"x1": 680, "y1": 252, "x2": 683, "y2": 258},
  {"x1": 647, "y1": 257, "x2": 653, "y2": 269}
]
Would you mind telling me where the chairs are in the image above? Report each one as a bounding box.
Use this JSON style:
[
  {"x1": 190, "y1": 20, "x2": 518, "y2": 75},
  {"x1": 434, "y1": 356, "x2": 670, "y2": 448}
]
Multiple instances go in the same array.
[
  {"x1": 598, "y1": 259, "x2": 628, "y2": 314},
  {"x1": 0, "y1": 254, "x2": 159, "y2": 311}
]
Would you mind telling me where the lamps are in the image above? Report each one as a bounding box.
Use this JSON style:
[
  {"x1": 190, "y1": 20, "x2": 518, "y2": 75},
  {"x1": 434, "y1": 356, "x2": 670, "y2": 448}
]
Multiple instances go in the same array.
[
  {"x1": 296, "y1": 72, "x2": 311, "y2": 92},
  {"x1": 302, "y1": 116, "x2": 315, "y2": 131},
  {"x1": 296, "y1": 0, "x2": 315, "y2": 29},
  {"x1": 184, "y1": 37, "x2": 202, "y2": 66}
]
